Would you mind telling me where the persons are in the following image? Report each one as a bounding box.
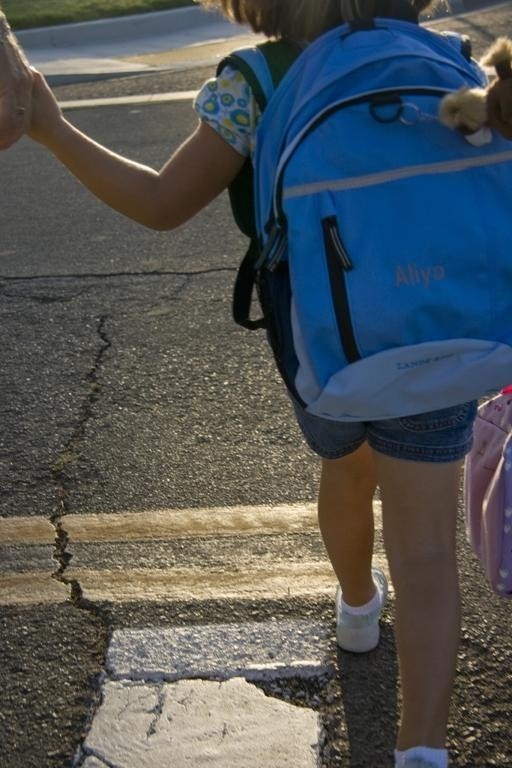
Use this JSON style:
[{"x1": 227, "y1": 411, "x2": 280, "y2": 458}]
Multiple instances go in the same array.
[
  {"x1": 24, "y1": 0, "x2": 512, "y2": 767},
  {"x1": 0, "y1": 5, "x2": 33, "y2": 151}
]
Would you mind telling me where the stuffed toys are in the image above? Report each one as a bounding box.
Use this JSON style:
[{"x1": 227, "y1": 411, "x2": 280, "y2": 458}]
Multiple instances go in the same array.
[{"x1": 438, "y1": 36, "x2": 511, "y2": 148}]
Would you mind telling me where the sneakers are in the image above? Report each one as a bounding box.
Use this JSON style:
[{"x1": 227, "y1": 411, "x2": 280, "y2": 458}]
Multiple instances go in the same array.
[{"x1": 335, "y1": 566, "x2": 388, "y2": 654}]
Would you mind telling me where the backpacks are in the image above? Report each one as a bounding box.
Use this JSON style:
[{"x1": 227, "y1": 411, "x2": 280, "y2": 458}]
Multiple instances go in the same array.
[{"x1": 214, "y1": 21, "x2": 511, "y2": 426}]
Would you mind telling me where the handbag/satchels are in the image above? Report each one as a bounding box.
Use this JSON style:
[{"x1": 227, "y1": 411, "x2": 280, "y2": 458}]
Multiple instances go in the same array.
[{"x1": 464, "y1": 383, "x2": 512, "y2": 601}]
